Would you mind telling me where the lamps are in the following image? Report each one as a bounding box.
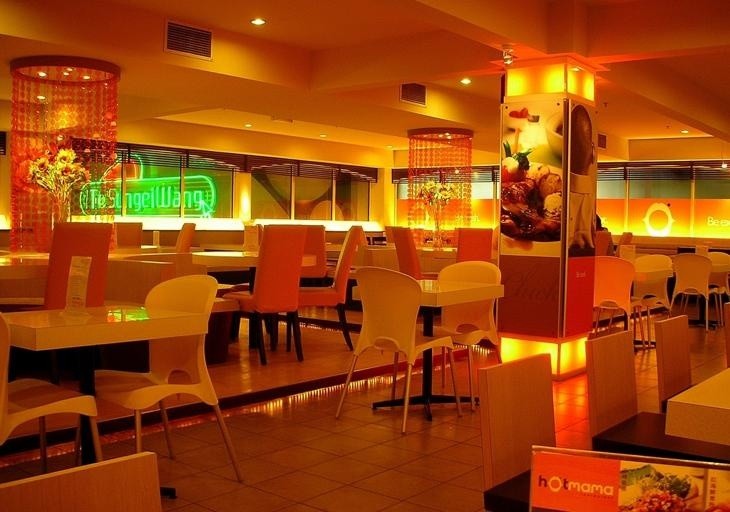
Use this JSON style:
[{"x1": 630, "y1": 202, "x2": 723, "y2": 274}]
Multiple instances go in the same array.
[
  {"x1": 406, "y1": 127, "x2": 474, "y2": 227},
  {"x1": 9, "y1": 53, "x2": 121, "y2": 252}
]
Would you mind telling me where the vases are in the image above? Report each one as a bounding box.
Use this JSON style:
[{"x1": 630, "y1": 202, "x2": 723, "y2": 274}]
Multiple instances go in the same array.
[{"x1": 51, "y1": 201, "x2": 71, "y2": 231}]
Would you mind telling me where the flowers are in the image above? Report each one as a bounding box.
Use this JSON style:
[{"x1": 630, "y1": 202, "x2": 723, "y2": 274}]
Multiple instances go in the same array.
[{"x1": 27, "y1": 148, "x2": 92, "y2": 198}]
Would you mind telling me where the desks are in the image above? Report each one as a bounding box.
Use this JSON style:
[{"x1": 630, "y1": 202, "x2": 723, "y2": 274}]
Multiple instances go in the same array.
[
  {"x1": 191, "y1": 251, "x2": 317, "y2": 350},
  {"x1": 624, "y1": 268, "x2": 674, "y2": 351},
  {"x1": 671, "y1": 263, "x2": 730, "y2": 330},
  {"x1": 3, "y1": 304, "x2": 208, "y2": 499},
  {"x1": 372, "y1": 279, "x2": 504, "y2": 421}
]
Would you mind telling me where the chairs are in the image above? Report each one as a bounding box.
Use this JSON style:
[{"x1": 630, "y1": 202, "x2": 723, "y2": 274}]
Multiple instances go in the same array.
[
  {"x1": 175, "y1": 223, "x2": 196, "y2": 252},
  {"x1": 223, "y1": 223, "x2": 308, "y2": 365},
  {"x1": 595, "y1": 232, "x2": 614, "y2": 256},
  {"x1": 593, "y1": 255, "x2": 635, "y2": 331},
  {"x1": 74, "y1": 274, "x2": 245, "y2": 484},
  {"x1": 114, "y1": 222, "x2": 142, "y2": 250},
  {"x1": 334, "y1": 267, "x2": 463, "y2": 435},
  {"x1": 1, "y1": 311, "x2": 106, "y2": 465},
  {"x1": 586, "y1": 333, "x2": 729, "y2": 463},
  {"x1": 393, "y1": 226, "x2": 439, "y2": 280},
  {"x1": 270, "y1": 225, "x2": 359, "y2": 352},
  {"x1": 655, "y1": 315, "x2": 693, "y2": 413},
  {"x1": 478, "y1": 352, "x2": 558, "y2": 512},
  {"x1": 18, "y1": 221, "x2": 113, "y2": 381},
  {"x1": 390, "y1": 261, "x2": 502, "y2": 414},
  {"x1": 456, "y1": 226, "x2": 493, "y2": 264},
  {"x1": 670, "y1": 253, "x2": 713, "y2": 333},
  {"x1": 300, "y1": 225, "x2": 326, "y2": 279},
  {"x1": 630, "y1": 255, "x2": 673, "y2": 350}
]
